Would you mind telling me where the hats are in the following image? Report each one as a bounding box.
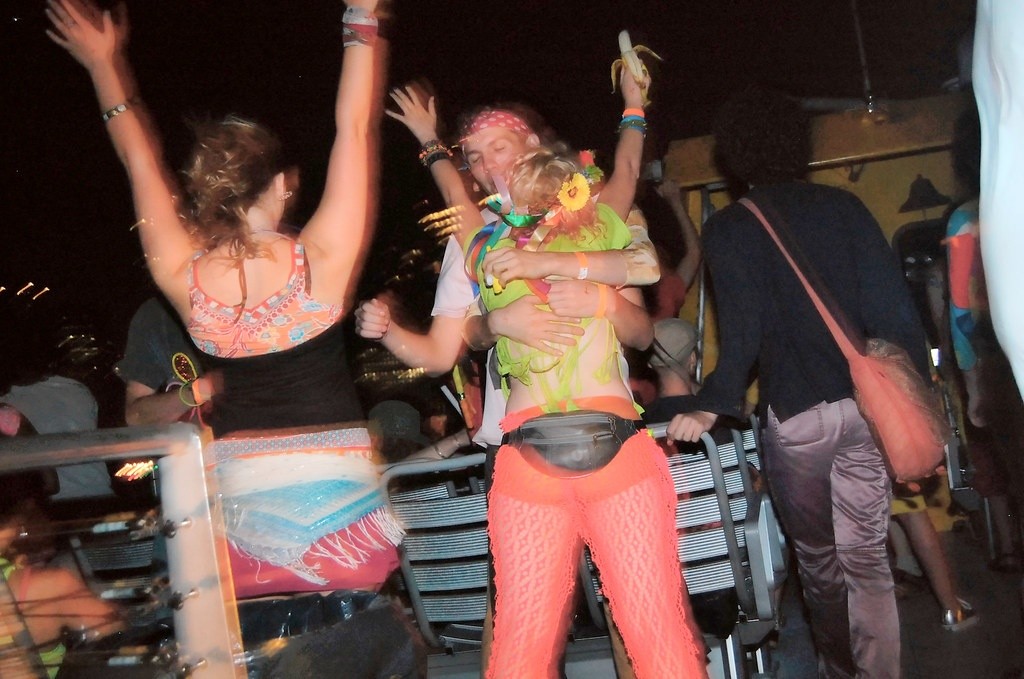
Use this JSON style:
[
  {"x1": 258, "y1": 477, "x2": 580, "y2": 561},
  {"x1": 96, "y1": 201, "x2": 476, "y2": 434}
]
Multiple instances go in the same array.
[{"x1": 647, "y1": 318, "x2": 696, "y2": 366}]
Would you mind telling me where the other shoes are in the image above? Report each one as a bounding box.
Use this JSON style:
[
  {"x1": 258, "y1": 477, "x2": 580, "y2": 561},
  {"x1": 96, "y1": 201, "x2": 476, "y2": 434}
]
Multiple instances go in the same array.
[
  {"x1": 991, "y1": 551, "x2": 1018, "y2": 573},
  {"x1": 941, "y1": 597, "x2": 979, "y2": 631}
]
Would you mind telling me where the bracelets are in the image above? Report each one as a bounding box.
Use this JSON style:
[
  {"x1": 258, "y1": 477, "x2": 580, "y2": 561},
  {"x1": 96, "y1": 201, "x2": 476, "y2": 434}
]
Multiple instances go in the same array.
[
  {"x1": 621, "y1": 107, "x2": 645, "y2": 118},
  {"x1": 420, "y1": 140, "x2": 454, "y2": 168},
  {"x1": 341, "y1": 7, "x2": 379, "y2": 47},
  {"x1": 101, "y1": 94, "x2": 142, "y2": 123},
  {"x1": 589, "y1": 281, "x2": 607, "y2": 319},
  {"x1": 620, "y1": 116, "x2": 647, "y2": 136},
  {"x1": 417, "y1": 144, "x2": 454, "y2": 166},
  {"x1": 573, "y1": 250, "x2": 589, "y2": 281},
  {"x1": 616, "y1": 120, "x2": 648, "y2": 133}
]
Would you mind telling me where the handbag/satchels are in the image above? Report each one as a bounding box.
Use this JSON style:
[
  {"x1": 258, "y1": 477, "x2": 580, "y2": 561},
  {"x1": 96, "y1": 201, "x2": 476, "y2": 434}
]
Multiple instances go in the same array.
[
  {"x1": 849, "y1": 337, "x2": 950, "y2": 492},
  {"x1": 500, "y1": 408, "x2": 646, "y2": 471}
]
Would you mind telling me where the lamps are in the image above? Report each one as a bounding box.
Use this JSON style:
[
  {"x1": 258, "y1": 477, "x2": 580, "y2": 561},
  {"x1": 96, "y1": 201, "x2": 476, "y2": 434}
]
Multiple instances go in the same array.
[{"x1": 898, "y1": 174, "x2": 951, "y2": 212}]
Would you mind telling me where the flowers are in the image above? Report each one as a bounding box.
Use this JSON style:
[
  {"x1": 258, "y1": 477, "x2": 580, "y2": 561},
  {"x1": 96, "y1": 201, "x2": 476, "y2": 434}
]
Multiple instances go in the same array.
[{"x1": 557, "y1": 148, "x2": 605, "y2": 212}]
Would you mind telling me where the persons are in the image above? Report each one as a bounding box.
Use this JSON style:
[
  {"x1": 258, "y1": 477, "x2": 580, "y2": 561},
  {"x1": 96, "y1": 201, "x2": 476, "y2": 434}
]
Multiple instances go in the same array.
[{"x1": 0, "y1": 0, "x2": 1024, "y2": 679}]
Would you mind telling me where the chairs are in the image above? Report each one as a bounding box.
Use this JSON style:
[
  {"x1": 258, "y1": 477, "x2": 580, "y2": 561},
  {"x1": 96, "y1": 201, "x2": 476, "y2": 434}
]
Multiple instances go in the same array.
[
  {"x1": 381, "y1": 413, "x2": 787, "y2": 679},
  {"x1": 0, "y1": 569, "x2": 52, "y2": 679},
  {"x1": 68, "y1": 527, "x2": 164, "y2": 605}
]
